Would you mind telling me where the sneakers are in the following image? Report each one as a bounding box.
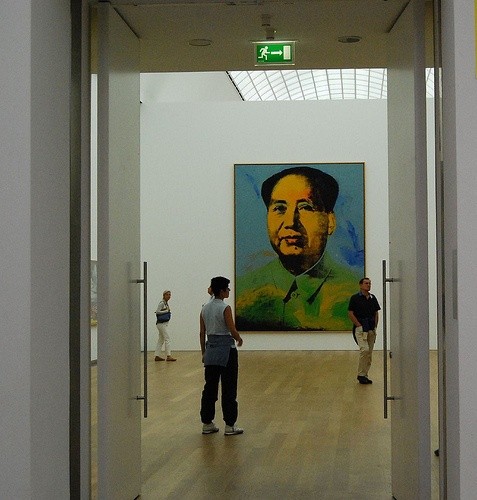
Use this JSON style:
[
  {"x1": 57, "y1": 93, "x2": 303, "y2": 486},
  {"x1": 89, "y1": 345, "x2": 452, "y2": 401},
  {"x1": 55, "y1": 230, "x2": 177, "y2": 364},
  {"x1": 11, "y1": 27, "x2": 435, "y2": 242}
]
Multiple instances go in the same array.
[
  {"x1": 202, "y1": 422, "x2": 219, "y2": 434},
  {"x1": 223, "y1": 425, "x2": 244, "y2": 436}
]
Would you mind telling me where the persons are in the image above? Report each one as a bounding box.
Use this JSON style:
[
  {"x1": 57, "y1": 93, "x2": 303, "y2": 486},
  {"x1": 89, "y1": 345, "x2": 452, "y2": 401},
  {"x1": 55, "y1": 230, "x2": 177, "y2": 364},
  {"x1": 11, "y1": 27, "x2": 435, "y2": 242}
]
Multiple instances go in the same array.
[
  {"x1": 200, "y1": 276, "x2": 245, "y2": 437},
  {"x1": 346, "y1": 277, "x2": 382, "y2": 385},
  {"x1": 201, "y1": 285, "x2": 215, "y2": 308},
  {"x1": 154, "y1": 289, "x2": 178, "y2": 361}
]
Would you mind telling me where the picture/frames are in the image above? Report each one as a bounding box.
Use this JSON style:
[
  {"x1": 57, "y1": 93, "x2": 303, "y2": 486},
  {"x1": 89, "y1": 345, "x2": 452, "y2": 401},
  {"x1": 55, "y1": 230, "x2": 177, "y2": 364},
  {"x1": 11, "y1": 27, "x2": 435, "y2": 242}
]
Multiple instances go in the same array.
[{"x1": 234, "y1": 163, "x2": 369, "y2": 335}]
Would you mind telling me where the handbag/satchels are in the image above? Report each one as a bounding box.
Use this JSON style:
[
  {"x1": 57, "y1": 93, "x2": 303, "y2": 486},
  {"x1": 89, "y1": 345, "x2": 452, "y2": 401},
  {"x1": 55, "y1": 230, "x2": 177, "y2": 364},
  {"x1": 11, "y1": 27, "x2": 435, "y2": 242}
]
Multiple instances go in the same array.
[{"x1": 153, "y1": 301, "x2": 170, "y2": 323}]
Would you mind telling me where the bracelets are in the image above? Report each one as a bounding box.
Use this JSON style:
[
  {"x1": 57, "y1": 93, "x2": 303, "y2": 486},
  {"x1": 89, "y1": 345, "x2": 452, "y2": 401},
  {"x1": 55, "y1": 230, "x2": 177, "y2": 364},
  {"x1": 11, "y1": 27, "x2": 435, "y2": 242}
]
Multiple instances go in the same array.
[{"x1": 239, "y1": 338, "x2": 242, "y2": 343}]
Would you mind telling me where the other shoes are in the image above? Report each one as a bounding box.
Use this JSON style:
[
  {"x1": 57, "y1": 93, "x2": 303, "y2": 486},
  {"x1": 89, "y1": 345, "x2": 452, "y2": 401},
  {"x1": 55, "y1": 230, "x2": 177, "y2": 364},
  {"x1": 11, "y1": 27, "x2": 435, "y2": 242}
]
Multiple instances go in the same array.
[
  {"x1": 364, "y1": 377, "x2": 372, "y2": 384},
  {"x1": 154, "y1": 356, "x2": 165, "y2": 361},
  {"x1": 166, "y1": 356, "x2": 177, "y2": 361},
  {"x1": 356, "y1": 375, "x2": 368, "y2": 384}
]
